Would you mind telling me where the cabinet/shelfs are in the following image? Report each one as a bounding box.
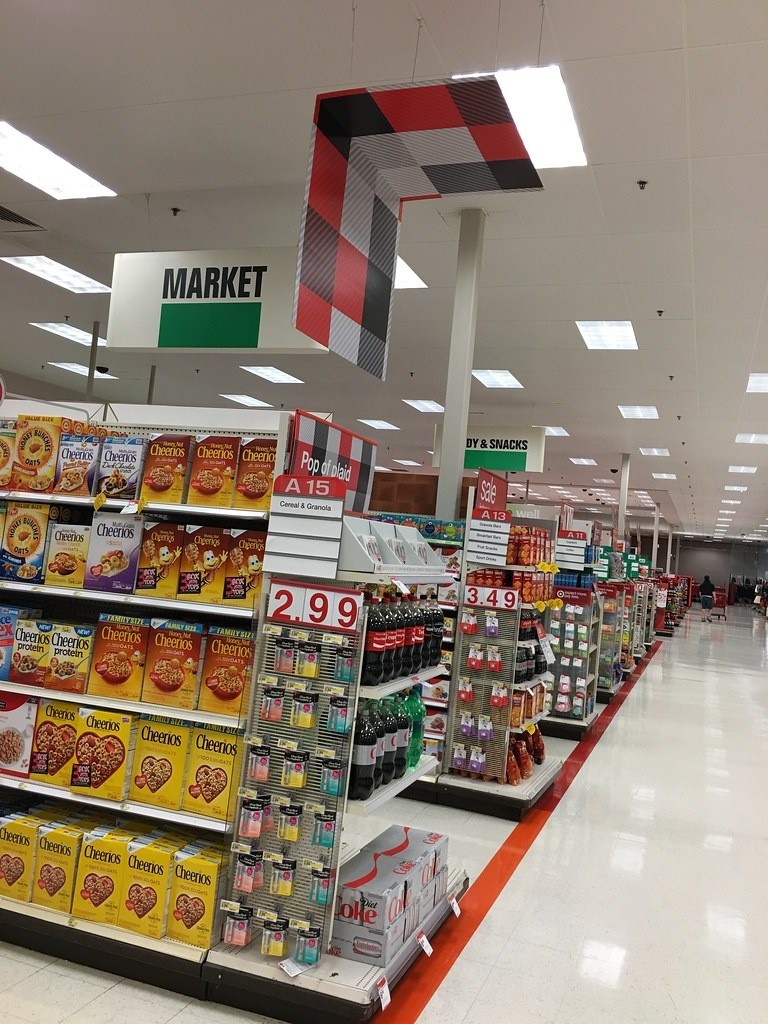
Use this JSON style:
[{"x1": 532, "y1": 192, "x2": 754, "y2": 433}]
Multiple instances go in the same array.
[
  {"x1": 356, "y1": 509, "x2": 564, "y2": 823},
  {"x1": 596, "y1": 511, "x2": 687, "y2": 705},
  {"x1": 536, "y1": 586, "x2": 606, "y2": 742},
  {"x1": 1, "y1": 397, "x2": 468, "y2": 1023}
]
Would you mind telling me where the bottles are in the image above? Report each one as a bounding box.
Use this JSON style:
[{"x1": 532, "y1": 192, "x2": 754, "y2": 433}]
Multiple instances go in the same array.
[
  {"x1": 359, "y1": 590, "x2": 444, "y2": 688},
  {"x1": 508, "y1": 610, "x2": 549, "y2": 683},
  {"x1": 348, "y1": 684, "x2": 426, "y2": 799},
  {"x1": 664, "y1": 618, "x2": 674, "y2": 630}
]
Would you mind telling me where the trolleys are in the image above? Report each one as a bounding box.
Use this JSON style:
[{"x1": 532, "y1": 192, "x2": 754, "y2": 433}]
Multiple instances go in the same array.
[{"x1": 710, "y1": 594, "x2": 726, "y2": 621}]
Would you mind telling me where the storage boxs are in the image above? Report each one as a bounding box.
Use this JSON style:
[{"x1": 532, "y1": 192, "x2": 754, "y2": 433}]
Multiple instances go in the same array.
[
  {"x1": 553, "y1": 545, "x2": 598, "y2": 592},
  {"x1": 367, "y1": 510, "x2": 464, "y2": 772},
  {"x1": 330, "y1": 824, "x2": 448, "y2": 967},
  {"x1": 0, "y1": 413, "x2": 280, "y2": 948},
  {"x1": 468, "y1": 523, "x2": 554, "y2": 603},
  {"x1": 509, "y1": 682, "x2": 546, "y2": 727}
]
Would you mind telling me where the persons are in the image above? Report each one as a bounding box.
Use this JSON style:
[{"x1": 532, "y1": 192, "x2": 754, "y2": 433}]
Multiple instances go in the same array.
[{"x1": 699, "y1": 576, "x2": 716, "y2": 622}]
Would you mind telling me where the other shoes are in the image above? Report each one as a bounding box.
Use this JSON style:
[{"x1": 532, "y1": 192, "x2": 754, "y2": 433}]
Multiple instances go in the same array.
[
  {"x1": 707, "y1": 617, "x2": 712, "y2": 622},
  {"x1": 702, "y1": 618, "x2": 706, "y2": 622}
]
